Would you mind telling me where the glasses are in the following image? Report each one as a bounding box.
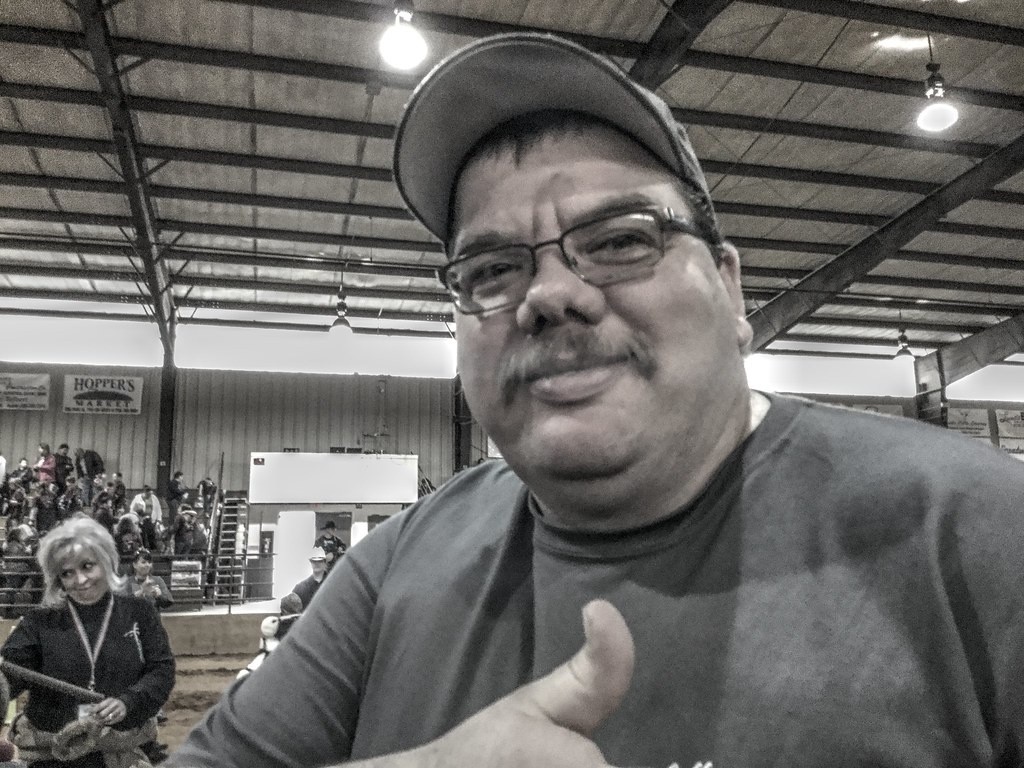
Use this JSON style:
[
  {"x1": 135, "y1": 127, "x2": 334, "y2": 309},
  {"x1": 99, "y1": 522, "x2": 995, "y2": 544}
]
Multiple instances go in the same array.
[{"x1": 434, "y1": 209, "x2": 716, "y2": 314}]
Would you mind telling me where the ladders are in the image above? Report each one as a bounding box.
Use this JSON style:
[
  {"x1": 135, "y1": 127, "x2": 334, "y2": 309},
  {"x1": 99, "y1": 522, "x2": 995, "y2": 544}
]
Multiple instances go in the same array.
[{"x1": 211, "y1": 490, "x2": 250, "y2": 604}]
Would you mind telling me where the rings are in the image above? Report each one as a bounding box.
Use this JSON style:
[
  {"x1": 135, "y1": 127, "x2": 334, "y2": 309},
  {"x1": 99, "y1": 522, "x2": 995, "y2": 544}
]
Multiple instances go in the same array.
[{"x1": 108, "y1": 714, "x2": 113, "y2": 720}]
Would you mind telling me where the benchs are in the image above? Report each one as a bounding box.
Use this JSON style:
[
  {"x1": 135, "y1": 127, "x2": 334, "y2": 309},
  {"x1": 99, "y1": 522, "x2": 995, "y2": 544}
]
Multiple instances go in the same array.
[{"x1": 2, "y1": 481, "x2": 213, "y2": 604}]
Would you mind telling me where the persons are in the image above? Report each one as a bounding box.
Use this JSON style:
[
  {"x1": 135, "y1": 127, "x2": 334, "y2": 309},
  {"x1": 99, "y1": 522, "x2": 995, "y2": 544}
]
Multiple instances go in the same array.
[
  {"x1": 170, "y1": 32, "x2": 1024, "y2": 768},
  {"x1": 0, "y1": 443, "x2": 350, "y2": 767}
]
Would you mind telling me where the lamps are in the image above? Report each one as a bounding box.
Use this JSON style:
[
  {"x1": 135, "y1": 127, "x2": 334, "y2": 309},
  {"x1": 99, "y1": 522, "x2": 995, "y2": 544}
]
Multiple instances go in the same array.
[
  {"x1": 916, "y1": 30, "x2": 958, "y2": 135},
  {"x1": 327, "y1": 268, "x2": 355, "y2": 344},
  {"x1": 379, "y1": 0, "x2": 428, "y2": 73}
]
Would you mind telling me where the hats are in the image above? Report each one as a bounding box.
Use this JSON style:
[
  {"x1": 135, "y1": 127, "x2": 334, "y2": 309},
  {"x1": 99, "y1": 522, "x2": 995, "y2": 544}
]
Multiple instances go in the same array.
[
  {"x1": 392, "y1": 34, "x2": 717, "y2": 258},
  {"x1": 320, "y1": 521, "x2": 337, "y2": 530},
  {"x1": 307, "y1": 546, "x2": 327, "y2": 561}
]
